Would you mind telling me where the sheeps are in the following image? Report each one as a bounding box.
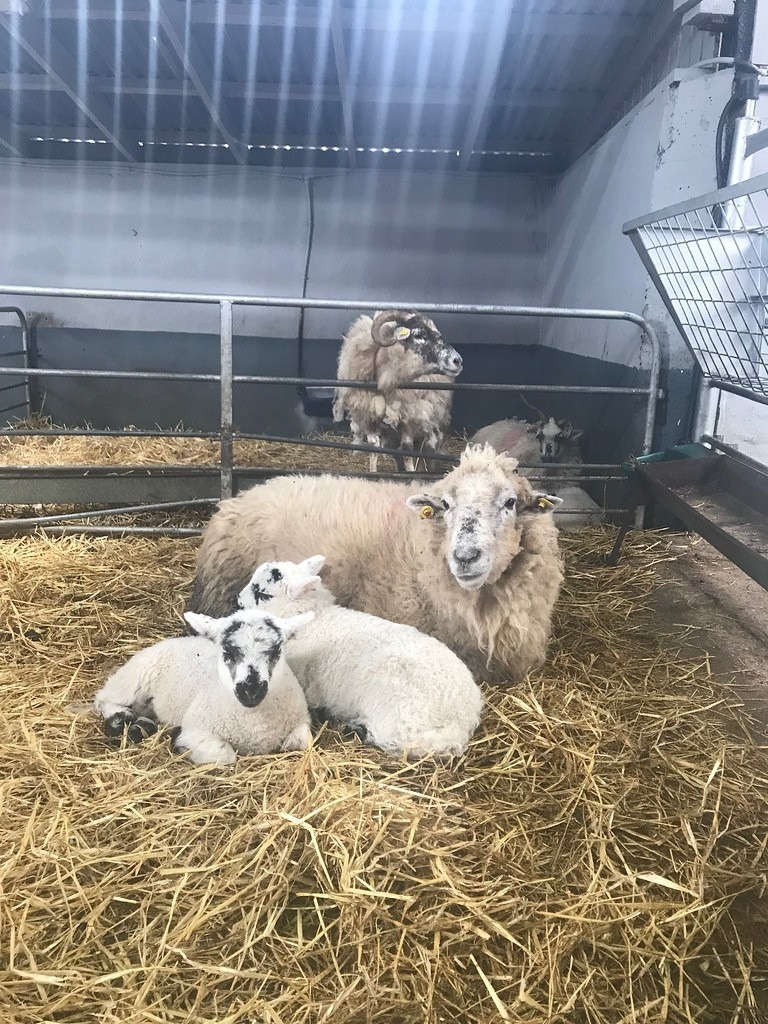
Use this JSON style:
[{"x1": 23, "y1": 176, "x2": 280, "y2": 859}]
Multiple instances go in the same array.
[
  {"x1": 235, "y1": 555, "x2": 483, "y2": 763},
  {"x1": 458, "y1": 414, "x2": 584, "y2": 488},
  {"x1": 332, "y1": 305, "x2": 463, "y2": 471},
  {"x1": 94, "y1": 608, "x2": 314, "y2": 767},
  {"x1": 185, "y1": 441, "x2": 565, "y2": 682}
]
